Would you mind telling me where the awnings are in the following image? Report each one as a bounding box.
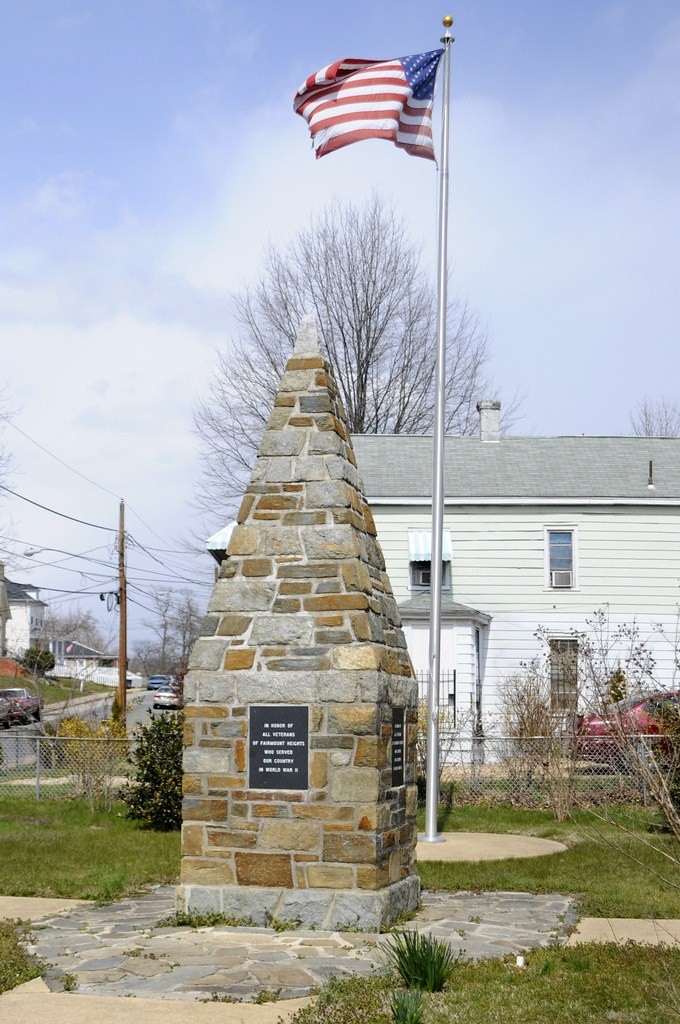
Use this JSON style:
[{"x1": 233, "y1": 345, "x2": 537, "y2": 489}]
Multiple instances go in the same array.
[
  {"x1": 408, "y1": 527, "x2": 453, "y2": 562},
  {"x1": 205, "y1": 521, "x2": 238, "y2": 566}
]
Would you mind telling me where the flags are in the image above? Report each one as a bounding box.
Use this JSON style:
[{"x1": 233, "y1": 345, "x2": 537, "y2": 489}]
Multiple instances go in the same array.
[{"x1": 293, "y1": 49, "x2": 447, "y2": 161}]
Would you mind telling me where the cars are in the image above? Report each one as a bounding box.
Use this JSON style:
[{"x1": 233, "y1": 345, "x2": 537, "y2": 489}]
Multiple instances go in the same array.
[
  {"x1": 576, "y1": 690, "x2": 680, "y2": 775},
  {"x1": 153, "y1": 686, "x2": 183, "y2": 710},
  {"x1": 147, "y1": 674, "x2": 177, "y2": 690}
]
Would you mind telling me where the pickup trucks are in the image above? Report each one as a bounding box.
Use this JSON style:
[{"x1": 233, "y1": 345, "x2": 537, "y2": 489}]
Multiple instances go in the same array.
[{"x1": 0, "y1": 688, "x2": 44, "y2": 729}]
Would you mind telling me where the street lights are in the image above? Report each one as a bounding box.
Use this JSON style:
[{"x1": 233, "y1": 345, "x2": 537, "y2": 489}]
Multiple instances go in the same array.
[{"x1": 24, "y1": 545, "x2": 127, "y2": 761}]
